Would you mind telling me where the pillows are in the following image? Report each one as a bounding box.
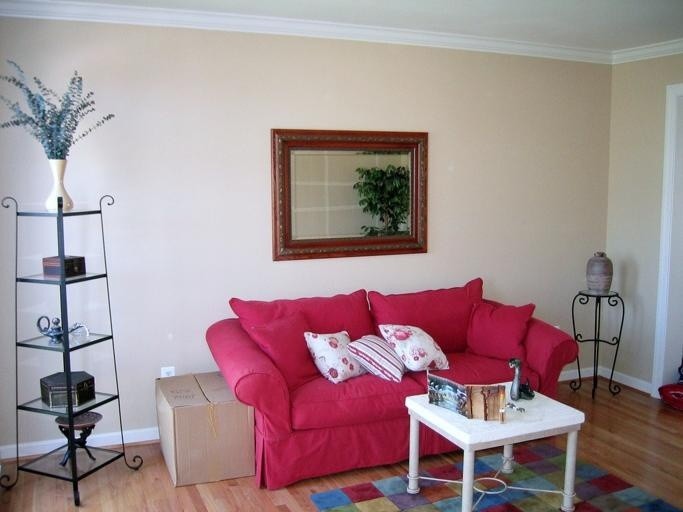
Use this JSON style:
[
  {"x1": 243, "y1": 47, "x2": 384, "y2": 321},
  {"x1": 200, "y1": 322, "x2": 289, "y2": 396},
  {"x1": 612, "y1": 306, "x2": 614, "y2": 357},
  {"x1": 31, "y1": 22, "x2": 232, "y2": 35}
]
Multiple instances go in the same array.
[
  {"x1": 368, "y1": 277, "x2": 483, "y2": 354},
  {"x1": 304, "y1": 330, "x2": 368, "y2": 383},
  {"x1": 378, "y1": 323, "x2": 450, "y2": 371},
  {"x1": 464, "y1": 298, "x2": 536, "y2": 366},
  {"x1": 346, "y1": 335, "x2": 406, "y2": 384},
  {"x1": 227, "y1": 288, "x2": 377, "y2": 387}
]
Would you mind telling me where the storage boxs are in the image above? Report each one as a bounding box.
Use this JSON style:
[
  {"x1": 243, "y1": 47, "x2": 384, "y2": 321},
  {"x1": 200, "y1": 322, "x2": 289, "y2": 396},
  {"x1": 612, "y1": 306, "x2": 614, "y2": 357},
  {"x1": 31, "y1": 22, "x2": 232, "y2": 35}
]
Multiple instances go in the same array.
[{"x1": 156, "y1": 372, "x2": 256, "y2": 489}]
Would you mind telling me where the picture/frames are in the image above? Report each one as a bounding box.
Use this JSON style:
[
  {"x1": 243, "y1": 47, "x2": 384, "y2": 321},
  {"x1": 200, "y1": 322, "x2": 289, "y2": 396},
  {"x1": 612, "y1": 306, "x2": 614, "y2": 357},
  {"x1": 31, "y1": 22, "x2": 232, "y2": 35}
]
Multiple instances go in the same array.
[{"x1": 270, "y1": 127, "x2": 431, "y2": 263}]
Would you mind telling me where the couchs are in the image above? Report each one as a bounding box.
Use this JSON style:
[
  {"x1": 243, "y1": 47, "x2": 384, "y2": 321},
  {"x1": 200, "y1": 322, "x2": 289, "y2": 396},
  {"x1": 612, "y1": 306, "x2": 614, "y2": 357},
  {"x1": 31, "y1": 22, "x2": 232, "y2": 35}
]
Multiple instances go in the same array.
[{"x1": 206, "y1": 276, "x2": 579, "y2": 491}]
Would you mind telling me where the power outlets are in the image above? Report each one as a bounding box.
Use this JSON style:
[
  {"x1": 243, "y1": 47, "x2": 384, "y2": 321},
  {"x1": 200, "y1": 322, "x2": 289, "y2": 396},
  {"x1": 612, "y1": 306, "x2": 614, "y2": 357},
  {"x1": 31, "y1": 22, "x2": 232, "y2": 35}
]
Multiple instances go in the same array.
[{"x1": 159, "y1": 366, "x2": 175, "y2": 378}]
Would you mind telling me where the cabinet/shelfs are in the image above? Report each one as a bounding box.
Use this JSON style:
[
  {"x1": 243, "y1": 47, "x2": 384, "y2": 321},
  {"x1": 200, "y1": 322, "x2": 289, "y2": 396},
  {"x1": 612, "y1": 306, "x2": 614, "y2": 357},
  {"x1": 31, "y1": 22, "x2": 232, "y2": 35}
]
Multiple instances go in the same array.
[{"x1": 1, "y1": 190, "x2": 144, "y2": 508}]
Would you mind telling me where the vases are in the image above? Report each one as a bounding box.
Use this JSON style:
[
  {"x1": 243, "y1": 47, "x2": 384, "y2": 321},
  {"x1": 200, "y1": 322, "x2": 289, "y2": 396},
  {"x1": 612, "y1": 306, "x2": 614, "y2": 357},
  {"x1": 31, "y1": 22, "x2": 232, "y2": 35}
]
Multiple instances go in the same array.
[{"x1": 43, "y1": 157, "x2": 75, "y2": 211}]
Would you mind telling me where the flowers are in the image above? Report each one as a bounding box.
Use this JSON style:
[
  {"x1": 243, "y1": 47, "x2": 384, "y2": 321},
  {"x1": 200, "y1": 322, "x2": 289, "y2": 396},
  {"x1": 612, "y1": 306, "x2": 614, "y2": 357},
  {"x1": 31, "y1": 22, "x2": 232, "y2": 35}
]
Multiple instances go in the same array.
[{"x1": 0, "y1": 59, "x2": 114, "y2": 160}]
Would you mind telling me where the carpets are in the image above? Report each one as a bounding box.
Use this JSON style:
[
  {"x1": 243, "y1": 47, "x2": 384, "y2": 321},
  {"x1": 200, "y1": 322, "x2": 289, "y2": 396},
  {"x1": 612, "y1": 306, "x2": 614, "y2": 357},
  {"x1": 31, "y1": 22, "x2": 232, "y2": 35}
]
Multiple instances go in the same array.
[{"x1": 308, "y1": 442, "x2": 683, "y2": 512}]
